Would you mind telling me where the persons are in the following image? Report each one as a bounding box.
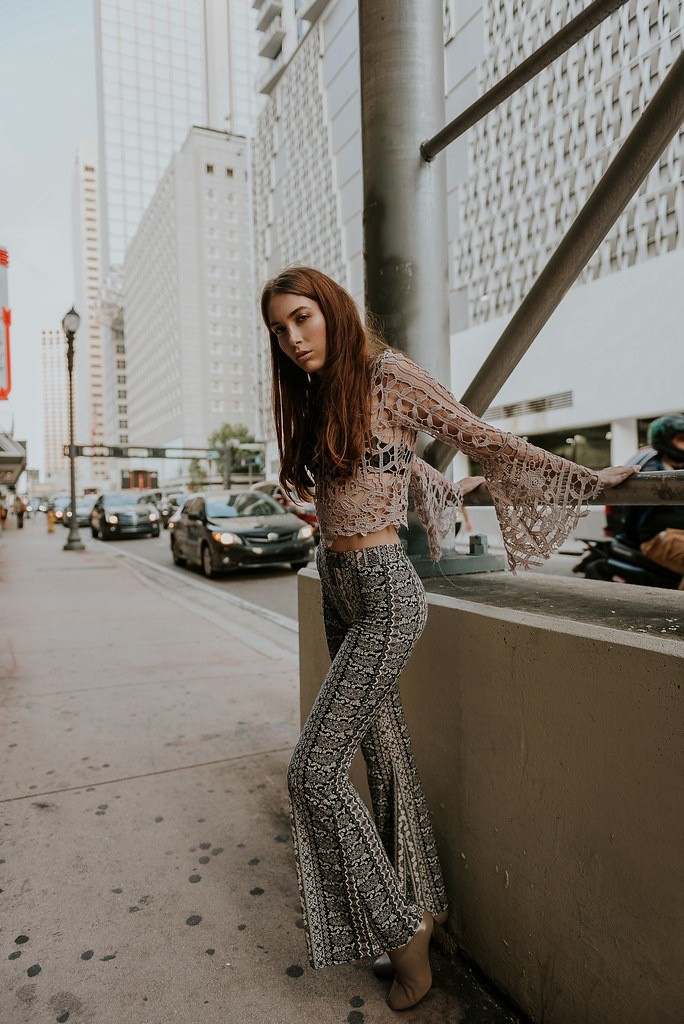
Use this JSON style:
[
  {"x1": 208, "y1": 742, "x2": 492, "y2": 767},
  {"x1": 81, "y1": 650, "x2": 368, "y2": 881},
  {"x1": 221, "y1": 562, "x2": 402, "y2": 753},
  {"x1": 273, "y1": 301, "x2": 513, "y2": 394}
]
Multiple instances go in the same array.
[
  {"x1": 0, "y1": 495, "x2": 10, "y2": 529},
  {"x1": 607, "y1": 412, "x2": 684, "y2": 590},
  {"x1": 14, "y1": 496, "x2": 27, "y2": 528},
  {"x1": 258, "y1": 266, "x2": 641, "y2": 1010}
]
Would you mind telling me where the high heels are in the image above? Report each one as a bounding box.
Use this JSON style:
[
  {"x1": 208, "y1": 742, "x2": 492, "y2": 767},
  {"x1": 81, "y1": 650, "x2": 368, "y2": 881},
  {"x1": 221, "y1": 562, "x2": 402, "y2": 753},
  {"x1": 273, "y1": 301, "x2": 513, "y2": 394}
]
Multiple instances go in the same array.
[
  {"x1": 373, "y1": 908, "x2": 451, "y2": 978},
  {"x1": 386, "y1": 908, "x2": 457, "y2": 1012}
]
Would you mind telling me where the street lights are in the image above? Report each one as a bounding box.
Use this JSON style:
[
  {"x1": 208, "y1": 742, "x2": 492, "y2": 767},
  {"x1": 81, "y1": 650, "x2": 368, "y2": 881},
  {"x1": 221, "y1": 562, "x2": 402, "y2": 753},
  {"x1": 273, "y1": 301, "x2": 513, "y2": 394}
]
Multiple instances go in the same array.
[{"x1": 61, "y1": 307, "x2": 89, "y2": 552}]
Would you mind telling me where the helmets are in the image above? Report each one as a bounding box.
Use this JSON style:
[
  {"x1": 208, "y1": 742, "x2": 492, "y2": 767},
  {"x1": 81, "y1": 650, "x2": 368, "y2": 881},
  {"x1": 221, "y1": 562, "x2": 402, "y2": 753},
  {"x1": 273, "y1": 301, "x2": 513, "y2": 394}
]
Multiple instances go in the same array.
[{"x1": 651, "y1": 414, "x2": 684, "y2": 462}]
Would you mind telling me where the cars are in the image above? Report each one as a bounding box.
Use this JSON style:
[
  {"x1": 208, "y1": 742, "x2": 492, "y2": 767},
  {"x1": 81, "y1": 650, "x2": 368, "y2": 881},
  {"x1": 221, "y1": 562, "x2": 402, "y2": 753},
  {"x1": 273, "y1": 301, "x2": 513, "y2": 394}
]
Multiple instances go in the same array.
[
  {"x1": 167, "y1": 491, "x2": 317, "y2": 579},
  {"x1": 137, "y1": 489, "x2": 192, "y2": 530},
  {"x1": 250, "y1": 480, "x2": 322, "y2": 541},
  {"x1": 62, "y1": 498, "x2": 97, "y2": 527},
  {"x1": 602, "y1": 448, "x2": 668, "y2": 540},
  {"x1": 28, "y1": 492, "x2": 73, "y2": 523},
  {"x1": 90, "y1": 492, "x2": 162, "y2": 541}
]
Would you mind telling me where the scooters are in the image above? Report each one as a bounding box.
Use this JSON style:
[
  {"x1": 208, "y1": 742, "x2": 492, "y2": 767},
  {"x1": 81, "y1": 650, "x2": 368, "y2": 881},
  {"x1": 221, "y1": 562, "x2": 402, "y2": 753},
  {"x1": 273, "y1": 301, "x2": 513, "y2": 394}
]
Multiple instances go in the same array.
[{"x1": 568, "y1": 530, "x2": 683, "y2": 589}]
